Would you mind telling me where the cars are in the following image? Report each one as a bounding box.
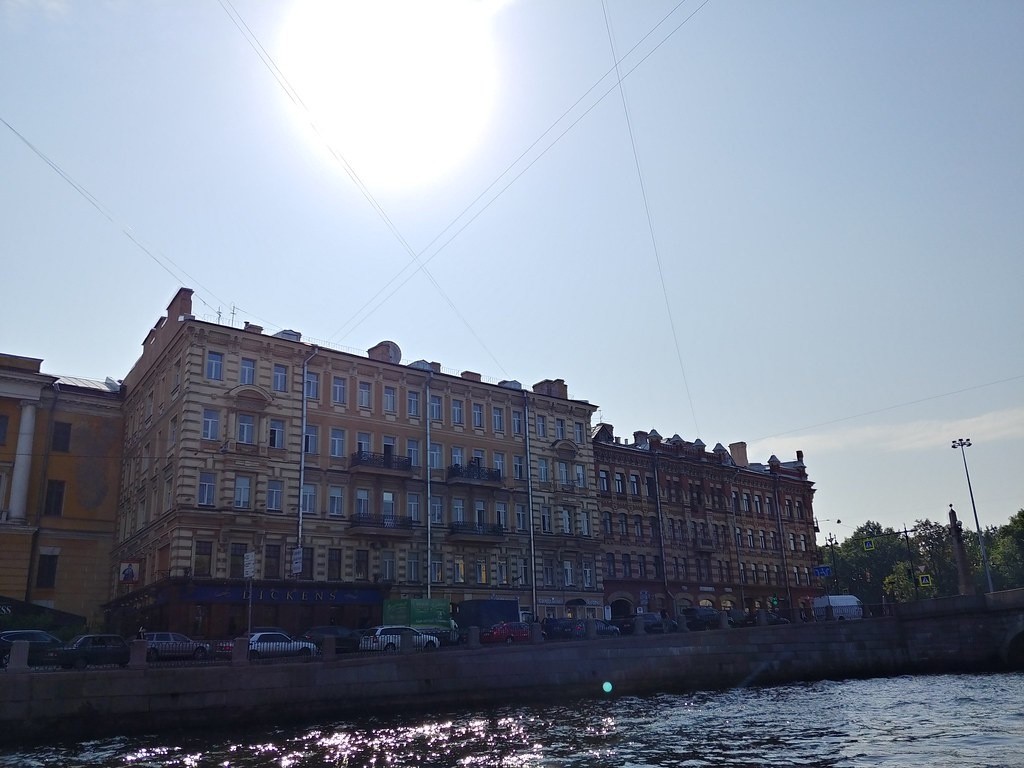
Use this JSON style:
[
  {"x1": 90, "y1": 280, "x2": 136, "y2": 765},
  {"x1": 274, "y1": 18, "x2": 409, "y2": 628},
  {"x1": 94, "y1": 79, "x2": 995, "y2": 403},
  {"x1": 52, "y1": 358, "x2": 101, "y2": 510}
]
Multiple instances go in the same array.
[
  {"x1": 743, "y1": 612, "x2": 791, "y2": 627},
  {"x1": 215, "y1": 632, "x2": 317, "y2": 659},
  {"x1": 143, "y1": 633, "x2": 211, "y2": 662},
  {"x1": 685, "y1": 608, "x2": 734, "y2": 631},
  {"x1": 562, "y1": 618, "x2": 621, "y2": 638},
  {"x1": 617, "y1": 612, "x2": 679, "y2": 634},
  {"x1": 44, "y1": 634, "x2": 131, "y2": 669},
  {"x1": 298, "y1": 626, "x2": 362, "y2": 653},
  {"x1": 191, "y1": 635, "x2": 235, "y2": 659},
  {"x1": 0, "y1": 630, "x2": 60, "y2": 669},
  {"x1": 359, "y1": 625, "x2": 439, "y2": 652},
  {"x1": 480, "y1": 621, "x2": 548, "y2": 643}
]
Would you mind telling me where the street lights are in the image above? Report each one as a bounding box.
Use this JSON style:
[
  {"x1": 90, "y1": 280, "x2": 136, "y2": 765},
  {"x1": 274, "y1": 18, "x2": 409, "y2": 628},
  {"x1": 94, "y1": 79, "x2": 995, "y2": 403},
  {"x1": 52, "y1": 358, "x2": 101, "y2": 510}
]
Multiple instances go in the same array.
[
  {"x1": 824, "y1": 532, "x2": 839, "y2": 595},
  {"x1": 952, "y1": 439, "x2": 994, "y2": 593}
]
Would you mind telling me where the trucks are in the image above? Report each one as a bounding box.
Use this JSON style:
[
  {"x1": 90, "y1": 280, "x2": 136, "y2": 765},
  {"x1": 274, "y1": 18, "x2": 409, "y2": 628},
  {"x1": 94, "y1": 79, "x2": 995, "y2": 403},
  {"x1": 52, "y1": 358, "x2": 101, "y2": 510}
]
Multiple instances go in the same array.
[{"x1": 814, "y1": 595, "x2": 873, "y2": 622}]
